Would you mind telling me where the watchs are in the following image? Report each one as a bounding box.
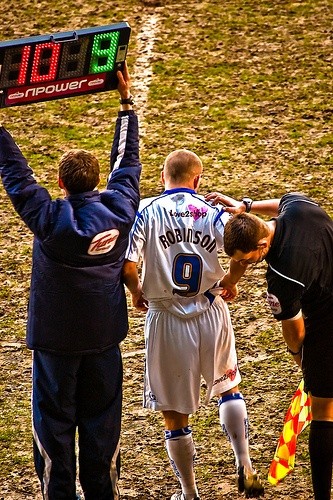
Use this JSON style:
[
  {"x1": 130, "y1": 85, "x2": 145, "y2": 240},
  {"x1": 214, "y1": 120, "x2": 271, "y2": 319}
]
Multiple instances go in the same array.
[
  {"x1": 120, "y1": 95, "x2": 134, "y2": 105},
  {"x1": 243, "y1": 197, "x2": 252, "y2": 213}
]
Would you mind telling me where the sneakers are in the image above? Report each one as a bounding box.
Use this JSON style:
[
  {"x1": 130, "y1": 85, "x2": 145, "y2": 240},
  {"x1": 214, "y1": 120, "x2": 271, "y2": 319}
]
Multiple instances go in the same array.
[
  {"x1": 171, "y1": 490, "x2": 201, "y2": 500},
  {"x1": 238, "y1": 466, "x2": 264, "y2": 497}
]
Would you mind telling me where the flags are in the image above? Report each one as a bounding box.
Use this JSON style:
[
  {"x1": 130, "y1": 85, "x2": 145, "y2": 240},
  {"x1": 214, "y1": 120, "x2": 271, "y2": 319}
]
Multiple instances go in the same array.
[{"x1": 268, "y1": 379, "x2": 311, "y2": 485}]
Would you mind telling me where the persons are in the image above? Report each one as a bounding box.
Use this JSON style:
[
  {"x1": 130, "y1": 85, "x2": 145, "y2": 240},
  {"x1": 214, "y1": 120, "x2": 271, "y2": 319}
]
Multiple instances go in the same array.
[
  {"x1": 204, "y1": 191, "x2": 333, "y2": 500},
  {"x1": 0, "y1": 60, "x2": 142, "y2": 500},
  {"x1": 123, "y1": 149, "x2": 265, "y2": 500}
]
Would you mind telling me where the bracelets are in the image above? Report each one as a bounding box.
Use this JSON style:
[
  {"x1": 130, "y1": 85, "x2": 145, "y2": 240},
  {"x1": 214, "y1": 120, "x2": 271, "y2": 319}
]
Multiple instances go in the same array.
[{"x1": 287, "y1": 346, "x2": 302, "y2": 355}]
